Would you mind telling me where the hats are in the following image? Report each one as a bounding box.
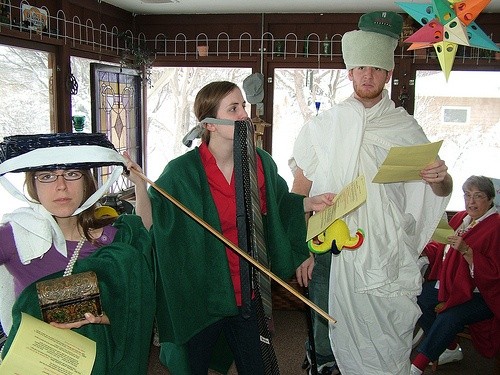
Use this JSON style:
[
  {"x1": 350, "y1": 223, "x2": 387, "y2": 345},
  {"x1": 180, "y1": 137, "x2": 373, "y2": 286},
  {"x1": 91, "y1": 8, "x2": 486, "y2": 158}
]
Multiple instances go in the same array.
[
  {"x1": 244, "y1": 73, "x2": 264, "y2": 104},
  {"x1": 359, "y1": 12, "x2": 403, "y2": 40},
  {"x1": 341, "y1": 30, "x2": 398, "y2": 71}
]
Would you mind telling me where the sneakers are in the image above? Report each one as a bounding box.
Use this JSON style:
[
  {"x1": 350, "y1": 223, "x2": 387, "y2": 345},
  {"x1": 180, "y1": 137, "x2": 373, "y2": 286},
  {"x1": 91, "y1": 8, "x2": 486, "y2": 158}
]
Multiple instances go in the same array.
[{"x1": 427, "y1": 344, "x2": 463, "y2": 365}]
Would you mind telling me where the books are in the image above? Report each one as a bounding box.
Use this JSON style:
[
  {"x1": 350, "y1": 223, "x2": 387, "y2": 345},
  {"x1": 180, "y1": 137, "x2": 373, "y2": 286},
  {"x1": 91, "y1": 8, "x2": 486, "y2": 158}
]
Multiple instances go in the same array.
[{"x1": 0, "y1": 312, "x2": 96, "y2": 375}]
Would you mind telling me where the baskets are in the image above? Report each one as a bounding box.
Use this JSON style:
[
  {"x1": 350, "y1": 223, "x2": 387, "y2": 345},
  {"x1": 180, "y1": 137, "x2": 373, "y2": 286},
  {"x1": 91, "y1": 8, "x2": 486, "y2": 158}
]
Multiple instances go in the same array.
[{"x1": 0, "y1": 132, "x2": 130, "y2": 178}]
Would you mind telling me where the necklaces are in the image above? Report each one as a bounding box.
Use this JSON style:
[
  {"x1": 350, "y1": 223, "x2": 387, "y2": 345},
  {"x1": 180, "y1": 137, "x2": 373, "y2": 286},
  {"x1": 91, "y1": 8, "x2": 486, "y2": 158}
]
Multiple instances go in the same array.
[{"x1": 62, "y1": 227, "x2": 92, "y2": 277}]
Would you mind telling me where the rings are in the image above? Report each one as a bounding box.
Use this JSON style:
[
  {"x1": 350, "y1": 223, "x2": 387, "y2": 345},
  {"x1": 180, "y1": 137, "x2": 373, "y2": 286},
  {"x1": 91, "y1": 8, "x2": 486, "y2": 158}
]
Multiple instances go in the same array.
[
  {"x1": 452, "y1": 242, "x2": 455, "y2": 246},
  {"x1": 436, "y1": 173, "x2": 439, "y2": 178}
]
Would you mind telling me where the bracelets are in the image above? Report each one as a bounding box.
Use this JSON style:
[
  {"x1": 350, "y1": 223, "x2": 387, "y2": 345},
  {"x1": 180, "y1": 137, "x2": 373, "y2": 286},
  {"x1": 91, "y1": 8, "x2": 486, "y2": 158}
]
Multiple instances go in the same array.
[
  {"x1": 462, "y1": 245, "x2": 471, "y2": 256},
  {"x1": 98, "y1": 315, "x2": 103, "y2": 325}
]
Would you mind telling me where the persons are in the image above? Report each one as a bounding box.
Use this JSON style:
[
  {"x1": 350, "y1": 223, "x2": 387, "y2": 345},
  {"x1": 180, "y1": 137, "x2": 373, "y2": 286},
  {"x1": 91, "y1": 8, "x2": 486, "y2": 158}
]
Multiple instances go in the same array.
[
  {"x1": 122, "y1": 81, "x2": 338, "y2": 375},
  {"x1": 288, "y1": 11, "x2": 453, "y2": 375},
  {"x1": 410, "y1": 176, "x2": 500, "y2": 375},
  {"x1": 0, "y1": 133, "x2": 156, "y2": 375}
]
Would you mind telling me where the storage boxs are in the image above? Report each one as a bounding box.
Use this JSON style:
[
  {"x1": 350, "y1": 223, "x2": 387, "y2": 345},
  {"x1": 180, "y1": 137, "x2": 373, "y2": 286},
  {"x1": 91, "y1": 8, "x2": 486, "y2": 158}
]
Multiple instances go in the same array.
[{"x1": 35, "y1": 271, "x2": 102, "y2": 324}]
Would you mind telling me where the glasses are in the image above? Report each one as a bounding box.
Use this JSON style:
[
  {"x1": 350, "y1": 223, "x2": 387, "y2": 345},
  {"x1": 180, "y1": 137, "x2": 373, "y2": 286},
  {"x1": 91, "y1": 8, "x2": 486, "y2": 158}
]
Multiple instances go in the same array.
[
  {"x1": 35, "y1": 170, "x2": 85, "y2": 183},
  {"x1": 464, "y1": 192, "x2": 489, "y2": 201}
]
